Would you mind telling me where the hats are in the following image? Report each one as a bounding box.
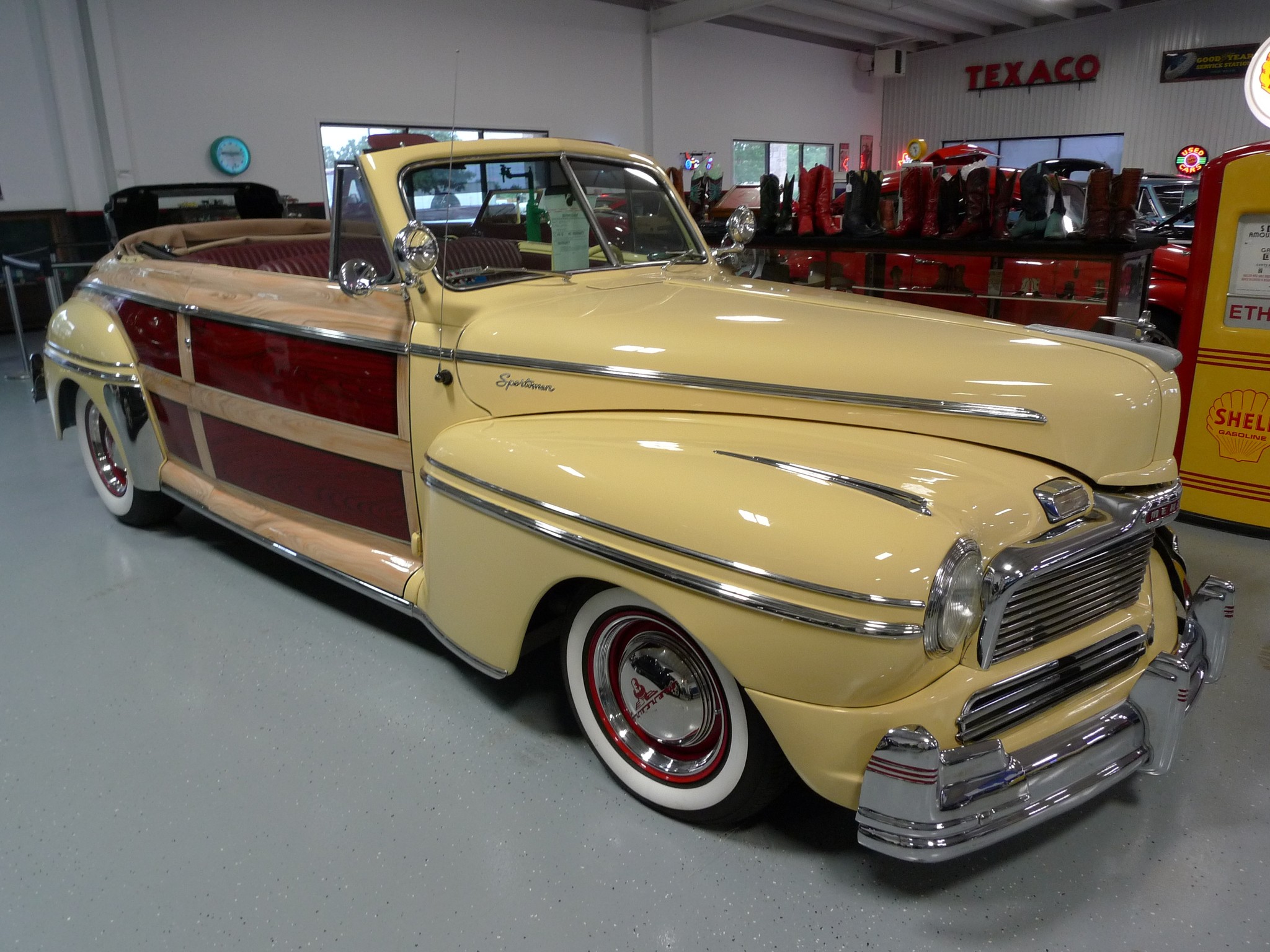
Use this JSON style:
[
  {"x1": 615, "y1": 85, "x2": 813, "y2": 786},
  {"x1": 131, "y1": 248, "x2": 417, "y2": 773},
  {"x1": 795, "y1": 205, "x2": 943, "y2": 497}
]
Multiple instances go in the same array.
[{"x1": 792, "y1": 261, "x2": 856, "y2": 287}]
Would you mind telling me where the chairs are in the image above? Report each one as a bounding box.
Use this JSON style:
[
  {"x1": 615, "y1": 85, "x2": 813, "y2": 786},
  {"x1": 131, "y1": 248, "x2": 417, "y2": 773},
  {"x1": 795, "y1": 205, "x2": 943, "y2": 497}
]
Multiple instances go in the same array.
[
  {"x1": 257, "y1": 251, "x2": 390, "y2": 285},
  {"x1": 431, "y1": 239, "x2": 524, "y2": 287}
]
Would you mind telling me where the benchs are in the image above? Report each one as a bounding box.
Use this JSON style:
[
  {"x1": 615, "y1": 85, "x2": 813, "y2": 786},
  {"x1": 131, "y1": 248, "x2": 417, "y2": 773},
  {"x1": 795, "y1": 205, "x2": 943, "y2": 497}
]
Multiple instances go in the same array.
[{"x1": 184, "y1": 236, "x2": 388, "y2": 269}]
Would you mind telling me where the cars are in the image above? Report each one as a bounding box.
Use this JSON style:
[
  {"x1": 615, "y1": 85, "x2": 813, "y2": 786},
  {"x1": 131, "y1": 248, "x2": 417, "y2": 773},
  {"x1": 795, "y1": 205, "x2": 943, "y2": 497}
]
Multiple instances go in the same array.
[
  {"x1": 588, "y1": 191, "x2": 676, "y2": 254},
  {"x1": 30, "y1": 138, "x2": 1240, "y2": 865},
  {"x1": 703, "y1": 170, "x2": 1200, "y2": 350},
  {"x1": 101, "y1": 181, "x2": 301, "y2": 250}
]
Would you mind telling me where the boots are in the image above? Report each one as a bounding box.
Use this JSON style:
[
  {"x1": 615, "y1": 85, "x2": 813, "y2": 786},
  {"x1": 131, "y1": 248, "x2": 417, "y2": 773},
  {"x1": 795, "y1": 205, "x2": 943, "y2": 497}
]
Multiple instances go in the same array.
[
  {"x1": 686, "y1": 170, "x2": 706, "y2": 227},
  {"x1": 797, "y1": 164, "x2": 823, "y2": 235},
  {"x1": 1012, "y1": 277, "x2": 1031, "y2": 298},
  {"x1": 927, "y1": 263, "x2": 949, "y2": 292},
  {"x1": 1115, "y1": 167, "x2": 1145, "y2": 244},
  {"x1": 1009, "y1": 175, "x2": 1048, "y2": 238},
  {"x1": 1044, "y1": 171, "x2": 1067, "y2": 240},
  {"x1": 815, "y1": 166, "x2": 844, "y2": 235},
  {"x1": 1067, "y1": 168, "x2": 1113, "y2": 240},
  {"x1": 661, "y1": 165, "x2": 685, "y2": 205},
  {"x1": 1055, "y1": 281, "x2": 1075, "y2": 300},
  {"x1": 885, "y1": 166, "x2": 922, "y2": 237},
  {"x1": 865, "y1": 170, "x2": 881, "y2": 230},
  {"x1": 889, "y1": 266, "x2": 903, "y2": 290},
  {"x1": 919, "y1": 166, "x2": 944, "y2": 237},
  {"x1": 1084, "y1": 279, "x2": 1105, "y2": 302},
  {"x1": 941, "y1": 166, "x2": 990, "y2": 242},
  {"x1": 991, "y1": 166, "x2": 1019, "y2": 242},
  {"x1": 1031, "y1": 277, "x2": 1042, "y2": 298},
  {"x1": 780, "y1": 173, "x2": 795, "y2": 225},
  {"x1": 704, "y1": 171, "x2": 724, "y2": 223},
  {"x1": 843, "y1": 171, "x2": 883, "y2": 238},
  {"x1": 952, "y1": 265, "x2": 970, "y2": 293},
  {"x1": 755, "y1": 173, "x2": 779, "y2": 235}
]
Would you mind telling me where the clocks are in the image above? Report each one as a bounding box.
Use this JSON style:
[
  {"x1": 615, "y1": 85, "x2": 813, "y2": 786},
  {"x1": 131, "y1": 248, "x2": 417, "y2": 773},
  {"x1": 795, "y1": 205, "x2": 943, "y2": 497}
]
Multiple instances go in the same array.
[{"x1": 211, "y1": 135, "x2": 252, "y2": 175}]
